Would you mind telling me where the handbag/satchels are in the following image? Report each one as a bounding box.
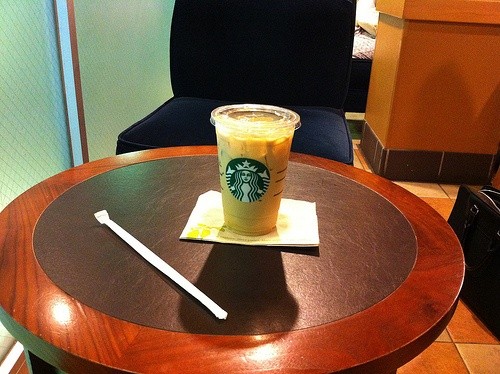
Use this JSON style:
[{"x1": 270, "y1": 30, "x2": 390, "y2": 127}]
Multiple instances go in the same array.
[{"x1": 447, "y1": 185, "x2": 500, "y2": 340}]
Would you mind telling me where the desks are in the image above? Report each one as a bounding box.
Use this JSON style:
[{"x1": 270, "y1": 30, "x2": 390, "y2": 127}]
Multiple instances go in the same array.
[{"x1": 0, "y1": 146, "x2": 466, "y2": 374}]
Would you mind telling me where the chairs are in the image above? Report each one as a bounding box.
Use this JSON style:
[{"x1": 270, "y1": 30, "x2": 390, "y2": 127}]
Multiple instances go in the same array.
[{"x1": 116, "y1": 0, "x2": 356, "y2": 165}]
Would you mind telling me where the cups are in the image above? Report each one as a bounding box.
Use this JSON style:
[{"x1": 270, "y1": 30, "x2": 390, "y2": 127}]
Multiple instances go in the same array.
[{"x1": 210, "y1": 104, "x2": 301, "y2": 234}]
